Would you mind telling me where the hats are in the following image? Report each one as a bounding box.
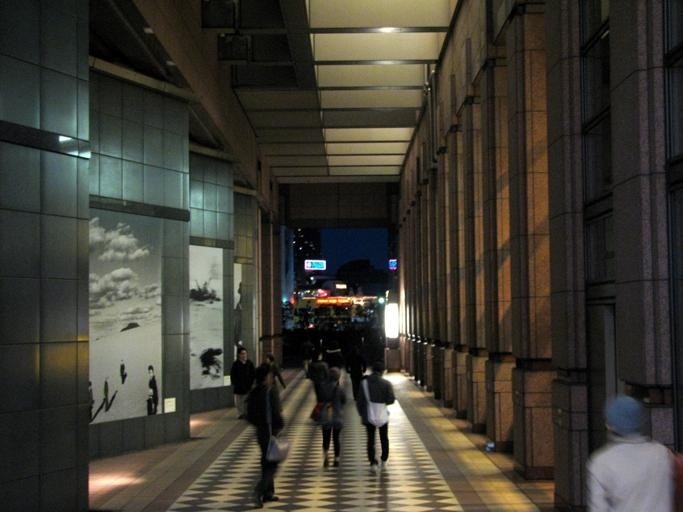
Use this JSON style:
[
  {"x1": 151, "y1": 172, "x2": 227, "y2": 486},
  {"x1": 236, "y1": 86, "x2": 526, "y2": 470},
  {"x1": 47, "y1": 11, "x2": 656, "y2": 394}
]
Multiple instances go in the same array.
[{"x1": 607, "y1": 395, "x2": 642, "y2": 433}]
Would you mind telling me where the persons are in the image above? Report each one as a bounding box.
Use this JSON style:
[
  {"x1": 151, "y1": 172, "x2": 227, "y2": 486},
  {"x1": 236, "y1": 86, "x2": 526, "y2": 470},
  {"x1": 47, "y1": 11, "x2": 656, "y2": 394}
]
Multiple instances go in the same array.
[
  {"x1": 253, "y1": 353, "x2": 286, "y2": 390},
  {"x1": 324, "y1": 340, "x2": 343, "y2": 385},
  {"x1": 305, "y1": 351, "x2": 329, "y2": 403},
  {"x1": 144, "y1": 365, "x2": 158, "y2": 415},
  {"x1": 243, "y1": 373, "x2": 285, "y2": 508},
  {"x1": 312, "y1": 365, "x2": 347, "y2": 467},
  {"x1": 581, "y1": 392, "x2": 681, "y2": 511},
  {"x1": 88, "y1": 360, "x2": 126, "y2": 422},
  {"x1": 357, "y1": 360, "x2": 395, "y2": 473},
  {"x1": 344, "y1": 345, "x2": 367, "y2": 401},
  {"x1": 198, "y1": 348, "x2": 222, "y2": 377},
  {"x1": 229, "y1": 347, "x2": 256, "y2": 420}
]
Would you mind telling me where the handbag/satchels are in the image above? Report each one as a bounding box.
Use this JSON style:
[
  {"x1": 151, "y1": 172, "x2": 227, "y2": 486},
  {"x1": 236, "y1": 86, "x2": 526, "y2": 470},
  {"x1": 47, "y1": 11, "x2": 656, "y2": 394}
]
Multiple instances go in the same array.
[
  {"x1": 367, "y1": 401, "x2": 389, "y2": 427},
  {"x1": 266, "y1": 435, "x2": 289, "y2": 461},
  {"x1": 311, "y1": 402, "x2": 334, "y2": 424}
]
